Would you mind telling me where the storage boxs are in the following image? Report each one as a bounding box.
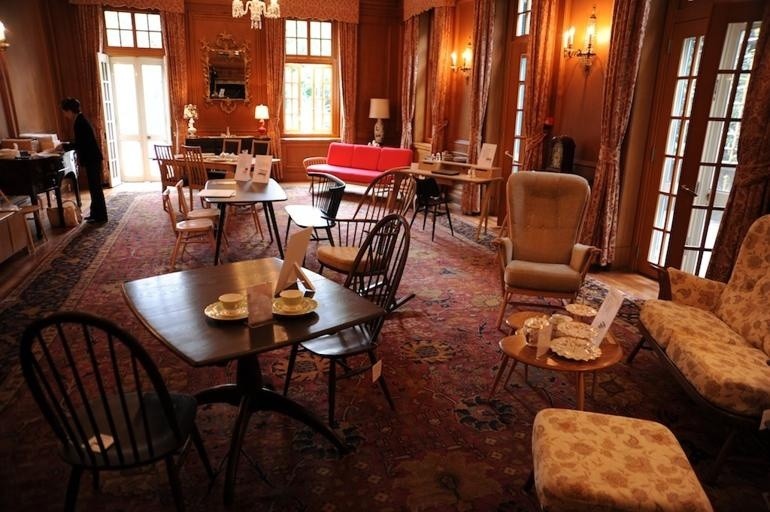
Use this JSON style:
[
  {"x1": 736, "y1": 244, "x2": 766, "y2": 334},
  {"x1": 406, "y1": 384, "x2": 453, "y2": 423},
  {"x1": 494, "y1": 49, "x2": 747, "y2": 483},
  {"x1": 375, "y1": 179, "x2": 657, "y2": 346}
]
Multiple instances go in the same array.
[{"x1": 1, "y1": 138, "x2": 40, "y2": 153}]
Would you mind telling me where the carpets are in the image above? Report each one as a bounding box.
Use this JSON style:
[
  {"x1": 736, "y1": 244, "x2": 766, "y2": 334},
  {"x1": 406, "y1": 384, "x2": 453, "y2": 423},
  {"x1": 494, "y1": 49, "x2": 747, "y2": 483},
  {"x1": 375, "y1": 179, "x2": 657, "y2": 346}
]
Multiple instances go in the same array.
[{"x1": 0, "y1": 182, "x2": 770, "y2": 512}]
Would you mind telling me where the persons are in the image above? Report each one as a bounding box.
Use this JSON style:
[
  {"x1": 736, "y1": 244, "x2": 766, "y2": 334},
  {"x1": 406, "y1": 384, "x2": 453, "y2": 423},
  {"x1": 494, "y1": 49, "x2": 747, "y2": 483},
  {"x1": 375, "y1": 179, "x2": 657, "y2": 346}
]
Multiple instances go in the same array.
[{"x1": 54, "y1": 96, "x2": 109, "y2": 224}]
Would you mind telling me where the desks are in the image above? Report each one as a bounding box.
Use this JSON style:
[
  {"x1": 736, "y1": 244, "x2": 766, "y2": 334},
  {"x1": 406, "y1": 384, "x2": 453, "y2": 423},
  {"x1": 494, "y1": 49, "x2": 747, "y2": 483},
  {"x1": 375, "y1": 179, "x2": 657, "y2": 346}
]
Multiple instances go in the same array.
[{"x1": 0, "y1": 153, "x2": 82, "y2": 239}]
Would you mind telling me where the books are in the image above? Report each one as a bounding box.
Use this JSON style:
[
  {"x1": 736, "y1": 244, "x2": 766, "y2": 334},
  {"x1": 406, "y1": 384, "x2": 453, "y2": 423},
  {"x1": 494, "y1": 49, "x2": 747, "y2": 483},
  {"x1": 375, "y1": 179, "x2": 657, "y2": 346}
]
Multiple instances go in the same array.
[{"x1": 198, "y1": 189, "x2": 237, "y2": 198}]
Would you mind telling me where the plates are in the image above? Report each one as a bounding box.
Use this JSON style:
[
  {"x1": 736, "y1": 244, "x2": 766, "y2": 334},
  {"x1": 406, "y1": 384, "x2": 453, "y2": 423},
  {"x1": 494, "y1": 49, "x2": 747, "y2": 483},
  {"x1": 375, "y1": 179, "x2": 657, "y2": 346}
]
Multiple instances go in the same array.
[
  {"x1": 549, "y1": 337, "x2": 602, "y2": 362},
  {"x1": 176, "y1": 153, "x2": 228, "y2": 162},
  {"x1": 564, "y1": 303, "x2": 597, "y2": 318},
  {"x1": 557, "y1": 322, "x2": 599, "y2": 339},
  {"x1": 204, "y1": 301, "x2": 254, "y2": 322},
  {"x1": 272, "y1": 297, "x2": 318, "y2": 317}
]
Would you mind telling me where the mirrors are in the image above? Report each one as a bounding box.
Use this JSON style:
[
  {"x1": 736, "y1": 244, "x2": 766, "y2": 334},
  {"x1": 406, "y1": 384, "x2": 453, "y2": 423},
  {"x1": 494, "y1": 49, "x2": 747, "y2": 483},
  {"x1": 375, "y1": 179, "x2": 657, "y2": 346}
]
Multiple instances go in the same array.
[{"x1": 201, "y1": 31, "x2": 253, "y2": 114}]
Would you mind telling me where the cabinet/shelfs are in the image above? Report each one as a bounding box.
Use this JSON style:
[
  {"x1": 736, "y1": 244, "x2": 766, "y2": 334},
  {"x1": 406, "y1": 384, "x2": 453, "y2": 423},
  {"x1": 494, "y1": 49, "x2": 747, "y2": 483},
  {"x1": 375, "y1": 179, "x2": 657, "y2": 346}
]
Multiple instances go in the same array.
[{"x1": 0, "y1": 208, "x2": 29, "y2": 272}]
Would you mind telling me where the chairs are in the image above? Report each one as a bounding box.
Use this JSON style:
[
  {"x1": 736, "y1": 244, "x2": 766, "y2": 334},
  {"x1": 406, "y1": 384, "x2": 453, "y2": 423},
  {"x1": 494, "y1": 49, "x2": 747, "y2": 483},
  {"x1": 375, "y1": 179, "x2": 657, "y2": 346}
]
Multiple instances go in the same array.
[{"x1": 0, "y1": 190, "x2": 49, "y2": 255}]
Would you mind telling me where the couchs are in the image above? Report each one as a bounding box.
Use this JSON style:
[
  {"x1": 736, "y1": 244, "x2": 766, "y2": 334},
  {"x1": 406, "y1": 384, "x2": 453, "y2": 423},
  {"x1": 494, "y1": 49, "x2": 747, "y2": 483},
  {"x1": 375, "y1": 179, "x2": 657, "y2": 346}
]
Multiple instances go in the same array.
[{"x1": 629, "y1": 215, "x2": 770, "y2": 481}]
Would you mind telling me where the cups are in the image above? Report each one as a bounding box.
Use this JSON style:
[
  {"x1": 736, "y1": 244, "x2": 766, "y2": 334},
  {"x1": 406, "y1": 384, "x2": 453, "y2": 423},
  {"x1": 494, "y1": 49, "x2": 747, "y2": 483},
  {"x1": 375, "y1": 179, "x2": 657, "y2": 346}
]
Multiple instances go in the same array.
[
  {"x1": 521, "y1": 315, "x2": 550, "y2": 349},
  {"x1": 549, "y1": 312, "x2": 564, "y2": 336},
  {"x1": 218, "y1": 292, "x2": 245, "y2": 314},
  {"x1": 278, "y1": 290, "x2": 304, "y2": 310}
]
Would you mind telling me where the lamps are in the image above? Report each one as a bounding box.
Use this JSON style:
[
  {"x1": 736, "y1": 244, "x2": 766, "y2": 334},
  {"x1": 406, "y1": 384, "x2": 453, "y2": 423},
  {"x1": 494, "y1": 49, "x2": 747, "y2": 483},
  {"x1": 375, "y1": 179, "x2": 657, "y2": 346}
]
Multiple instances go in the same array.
[
  {"x1": 183, "y1": 105, "x2": 200, "y2": 139},
  {"x1": 450, "y1": 47, "x2": 474, "y2": 73},
  {"x1": 369, "y1": 98, "x2": 390, "y2": 144},
  {"x1": 232, "y1": 0, "x2": 281, "y2": 31},
  {"x1": 255, "y1": 106, "x2": 270, "y2": 134},
  {"x1": 0, "y1": 20, "x2": 10, "y2": 51},
  {"x1": 564, "y1": 15, "x2": 602, "y2": 72}
]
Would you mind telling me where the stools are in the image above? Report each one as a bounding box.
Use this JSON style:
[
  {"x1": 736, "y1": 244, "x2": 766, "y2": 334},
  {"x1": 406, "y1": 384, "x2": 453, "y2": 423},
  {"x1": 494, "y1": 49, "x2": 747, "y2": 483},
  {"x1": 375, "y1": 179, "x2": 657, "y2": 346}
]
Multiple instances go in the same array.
[{"x1": 531, "y1": 407, "x2": 714, "y2": 512}]
